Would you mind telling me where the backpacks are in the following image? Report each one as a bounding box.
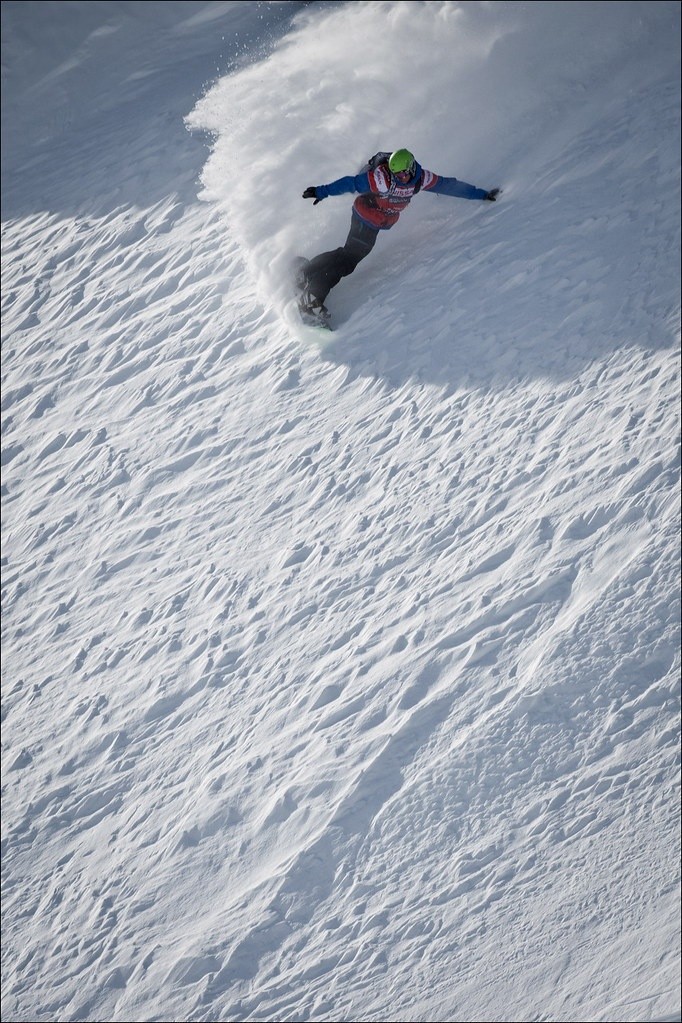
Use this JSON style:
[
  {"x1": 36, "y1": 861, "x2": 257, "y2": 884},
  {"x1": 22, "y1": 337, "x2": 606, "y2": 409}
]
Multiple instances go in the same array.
[{"x1": 369, "y1": 151, "x2": 422, "y2": 197}]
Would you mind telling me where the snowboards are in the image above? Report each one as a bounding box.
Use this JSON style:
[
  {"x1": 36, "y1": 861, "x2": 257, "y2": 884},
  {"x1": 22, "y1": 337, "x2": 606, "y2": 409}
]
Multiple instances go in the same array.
[{"x1": 286, "y1": 258, "x2": 332, "y2": 332}]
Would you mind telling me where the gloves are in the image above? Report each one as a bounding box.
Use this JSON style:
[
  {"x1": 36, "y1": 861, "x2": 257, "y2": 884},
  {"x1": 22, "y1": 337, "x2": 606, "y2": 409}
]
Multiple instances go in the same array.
[
  {"x1": 302, "y1": 187, "x2": 320, "y2": 205},
  {"x1": 483, "y1": 188, "x2": 499, "y2": 201}
]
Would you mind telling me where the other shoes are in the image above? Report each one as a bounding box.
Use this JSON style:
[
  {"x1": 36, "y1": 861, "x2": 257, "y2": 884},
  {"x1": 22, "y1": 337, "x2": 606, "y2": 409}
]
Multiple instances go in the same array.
[
  {"x1": 297, "y1": 291, "x2": 331, "y2": 320},
  {"x1": 292, "y1": 269, "x2": 311, "y2": 292}
]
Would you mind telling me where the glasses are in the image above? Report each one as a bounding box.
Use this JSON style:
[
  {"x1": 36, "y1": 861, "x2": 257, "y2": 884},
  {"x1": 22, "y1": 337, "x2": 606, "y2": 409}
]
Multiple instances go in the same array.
[{"x1": 394, "y1": 171, "x2": 408, "y2": 179}]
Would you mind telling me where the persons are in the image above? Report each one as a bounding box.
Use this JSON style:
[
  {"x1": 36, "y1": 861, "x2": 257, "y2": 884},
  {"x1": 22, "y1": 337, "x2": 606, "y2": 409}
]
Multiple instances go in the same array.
[{"x1": 293, "y1": 149, "x2": 500, "y2": 319}]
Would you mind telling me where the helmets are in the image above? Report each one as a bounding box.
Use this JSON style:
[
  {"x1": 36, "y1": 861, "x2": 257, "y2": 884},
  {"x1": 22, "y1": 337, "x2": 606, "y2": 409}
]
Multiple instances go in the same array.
[{"x1": 388, "y1": 148, "x2": 414, "y2": 174}]
